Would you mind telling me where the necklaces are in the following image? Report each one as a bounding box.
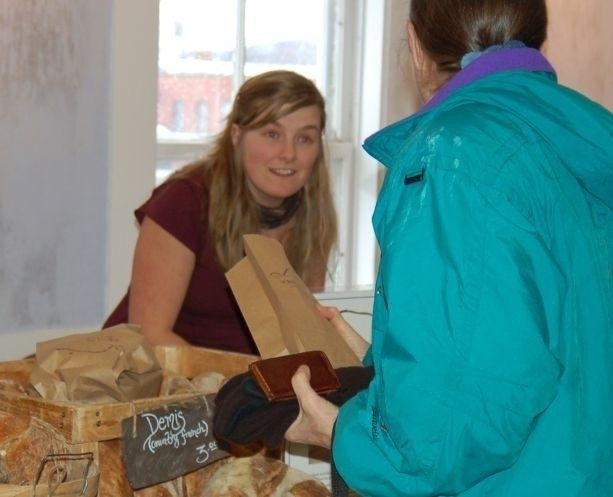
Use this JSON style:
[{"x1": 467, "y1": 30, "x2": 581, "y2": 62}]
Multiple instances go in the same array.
[{"x1": 260, "y1": 191, "x2": 306, "y2": 230}]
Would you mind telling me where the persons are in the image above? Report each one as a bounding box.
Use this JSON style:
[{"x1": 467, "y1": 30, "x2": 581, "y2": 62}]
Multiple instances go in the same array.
[
  {"x1": 282, "y1": 0, "x2": 613, "y2": 496},
  {"x1": 103, "y1": 71, "x2": 334, "y2": 350}
]
